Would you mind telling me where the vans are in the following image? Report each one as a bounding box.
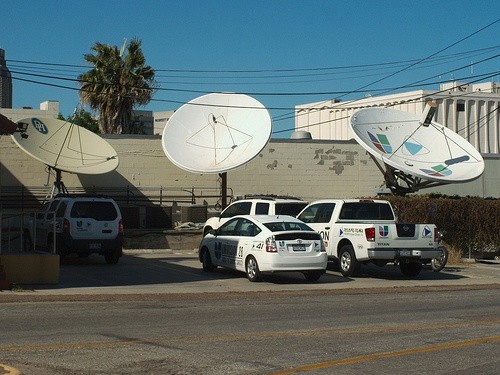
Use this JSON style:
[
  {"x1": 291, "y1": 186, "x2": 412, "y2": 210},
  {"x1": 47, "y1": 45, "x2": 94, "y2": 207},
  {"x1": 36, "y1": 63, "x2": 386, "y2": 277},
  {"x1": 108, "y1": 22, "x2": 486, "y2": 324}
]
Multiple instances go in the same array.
[{"x1": 22, "y1": 193, "x2": 124, "y2": 265}]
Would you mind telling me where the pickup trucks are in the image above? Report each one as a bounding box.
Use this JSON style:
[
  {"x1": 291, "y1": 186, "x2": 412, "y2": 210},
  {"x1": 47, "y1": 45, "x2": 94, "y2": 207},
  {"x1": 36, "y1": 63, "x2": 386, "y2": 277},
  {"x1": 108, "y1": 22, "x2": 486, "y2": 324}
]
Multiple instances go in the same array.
[{"x1": 295, "y1": 199, "x2": 443, "y2": 276}]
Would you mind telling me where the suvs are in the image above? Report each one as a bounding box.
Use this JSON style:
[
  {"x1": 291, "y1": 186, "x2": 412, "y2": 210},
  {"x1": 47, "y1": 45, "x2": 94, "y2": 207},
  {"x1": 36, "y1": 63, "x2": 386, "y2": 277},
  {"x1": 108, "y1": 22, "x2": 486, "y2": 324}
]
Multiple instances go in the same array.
[{"x1": 202, "y1": 198, "x2": 315, "y2": 236}]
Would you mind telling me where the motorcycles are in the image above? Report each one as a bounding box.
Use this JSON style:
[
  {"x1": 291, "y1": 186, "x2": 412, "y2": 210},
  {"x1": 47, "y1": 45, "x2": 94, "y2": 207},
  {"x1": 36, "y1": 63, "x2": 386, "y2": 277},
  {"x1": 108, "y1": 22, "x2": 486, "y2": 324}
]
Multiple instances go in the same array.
[{"x1": 392, "y1": 231, "x2": 449, "y2": 272}]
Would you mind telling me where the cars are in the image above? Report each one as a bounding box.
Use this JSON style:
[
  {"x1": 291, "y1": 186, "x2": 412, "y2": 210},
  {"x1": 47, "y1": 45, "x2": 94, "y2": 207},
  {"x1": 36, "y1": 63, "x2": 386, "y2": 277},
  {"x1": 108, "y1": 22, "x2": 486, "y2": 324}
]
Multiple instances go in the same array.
[{"x1": 198, "y1": 215, "x2": 328, "y2": 283}]
